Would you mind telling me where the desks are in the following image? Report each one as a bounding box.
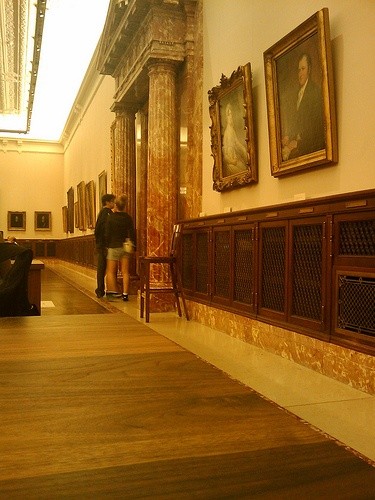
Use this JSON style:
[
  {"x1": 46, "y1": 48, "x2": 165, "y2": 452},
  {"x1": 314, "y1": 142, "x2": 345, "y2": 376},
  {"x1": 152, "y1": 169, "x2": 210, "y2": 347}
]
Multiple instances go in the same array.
[{"x1": 11, "y1": 259, "x2": 45, "y2": 316}]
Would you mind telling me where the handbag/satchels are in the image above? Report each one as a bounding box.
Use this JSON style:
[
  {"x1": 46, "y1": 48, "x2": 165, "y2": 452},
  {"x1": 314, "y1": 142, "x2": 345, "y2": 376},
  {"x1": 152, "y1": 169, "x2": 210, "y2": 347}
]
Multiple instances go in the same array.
[{"x1": 124, "y1": 237, "x2": 135, "y2": 254}]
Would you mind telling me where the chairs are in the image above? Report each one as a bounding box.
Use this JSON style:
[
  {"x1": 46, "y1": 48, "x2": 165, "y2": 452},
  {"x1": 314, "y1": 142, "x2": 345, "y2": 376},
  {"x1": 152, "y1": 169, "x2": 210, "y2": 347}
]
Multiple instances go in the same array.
[{"x1": 138, "y1": 224, "x2": 189, "y2": 324}]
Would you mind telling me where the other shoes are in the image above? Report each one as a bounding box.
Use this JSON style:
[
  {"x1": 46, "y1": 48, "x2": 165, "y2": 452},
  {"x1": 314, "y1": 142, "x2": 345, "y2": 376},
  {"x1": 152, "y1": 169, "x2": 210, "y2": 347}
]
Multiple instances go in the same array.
[
  {"x1": 106, "y1": 290, "x2": 122, "y2": 297},
  {"x1": 122, "y1": 293, "x2": 128, "y2": 301},
  {"x1": 95, "y1": 290, "x2": 105, "y2": 297}
]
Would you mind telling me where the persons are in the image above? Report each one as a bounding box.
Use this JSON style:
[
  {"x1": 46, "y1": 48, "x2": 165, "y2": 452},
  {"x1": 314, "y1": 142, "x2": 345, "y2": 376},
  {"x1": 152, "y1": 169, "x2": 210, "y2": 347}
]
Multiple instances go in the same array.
[
  {"x1": 104, "y1": 194, "x2": 136, "y2": 302},
  {"x1": 0, "y1": 237, "x2": 39, "y2": 314},
  {"x1": 95, "y1": 193, "x2": 115, "y2": 298}
]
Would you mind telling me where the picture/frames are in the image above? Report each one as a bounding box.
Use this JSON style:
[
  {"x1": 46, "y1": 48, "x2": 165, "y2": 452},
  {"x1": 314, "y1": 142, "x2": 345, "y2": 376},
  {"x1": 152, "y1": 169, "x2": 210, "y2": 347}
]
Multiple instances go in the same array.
[
  {"x1": 62, "y1": 170, "x2": 108, "y2": 233},
  {"x1": 34, "y1": 211, "x2": 52, "y2": 231},
  {"x1": 208, "y1": 62, "x2": 259, "y2": 192},
  {"x1": 263, "y1": 8, "x2": 339, "y2": 178},
  {"x1": 8, "y1": 211, "x2": 26, "y2": 231}
]
